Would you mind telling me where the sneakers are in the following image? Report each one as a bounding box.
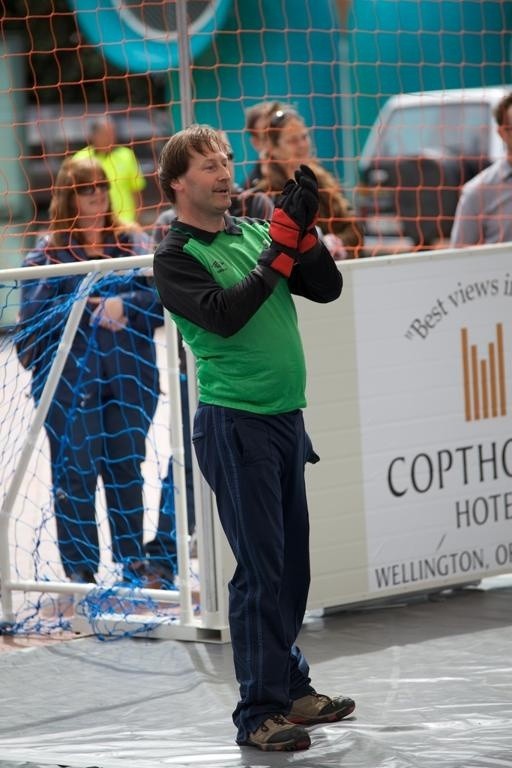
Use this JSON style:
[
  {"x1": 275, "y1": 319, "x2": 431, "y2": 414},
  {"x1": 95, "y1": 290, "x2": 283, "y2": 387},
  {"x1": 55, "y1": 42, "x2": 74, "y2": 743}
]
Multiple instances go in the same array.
[
  {"x1": 244, "y1": 712, "x2": 312, "y2": 751},
  {"x1": 67, "y1": 562, "x2": 174, "y2": 596},
  {"x1": 285, "y1": 689, "x2": 357, "y2": 726}
]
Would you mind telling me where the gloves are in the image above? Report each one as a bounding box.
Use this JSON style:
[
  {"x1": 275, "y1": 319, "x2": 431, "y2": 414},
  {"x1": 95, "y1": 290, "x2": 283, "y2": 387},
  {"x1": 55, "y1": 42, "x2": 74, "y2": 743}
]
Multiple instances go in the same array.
[
  {"x1": 291, "y1": 163, "x2": 320, "y2": 256},
  {"x1": 256, "y1": 183, "x2": 310, "y2": 281}
]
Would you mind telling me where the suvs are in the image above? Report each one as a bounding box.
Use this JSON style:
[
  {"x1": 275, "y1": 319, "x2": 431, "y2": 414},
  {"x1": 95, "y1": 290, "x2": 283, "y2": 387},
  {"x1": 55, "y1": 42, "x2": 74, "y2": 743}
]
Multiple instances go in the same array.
[{"x1": 352, "y1": 85, "x2": 510, "y2": 255}]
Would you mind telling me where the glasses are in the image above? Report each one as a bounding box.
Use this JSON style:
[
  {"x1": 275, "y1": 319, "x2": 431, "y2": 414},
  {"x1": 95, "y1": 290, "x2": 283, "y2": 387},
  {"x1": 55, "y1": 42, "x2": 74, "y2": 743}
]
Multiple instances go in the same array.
[
  {"x1": 75, "y1": 180, "x2": 112, "y2": 196},
  {"x1": 498, "y1": 123, "x2": 511, "y2": 143}
]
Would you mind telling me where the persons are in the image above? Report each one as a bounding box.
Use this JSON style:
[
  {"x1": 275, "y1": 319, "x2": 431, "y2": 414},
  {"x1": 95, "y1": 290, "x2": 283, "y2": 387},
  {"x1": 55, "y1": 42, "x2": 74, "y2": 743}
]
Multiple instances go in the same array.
[
  {"x1": 143, "y1": 129, "x2": 275, "y2": 591},
  {"x1": 449, "y1": 92, "x2": 512, "y2": 248},
  {"x1": 76, "y1": 112, "x2": 147, "y2": 223},
  {"x1": 149, "y1": 123, "x2": 356, "y2": 747},
  {"x1": 238, "y1": 100, "x2": 364, "y2": 261},
  {"x1": 14, "y1": 155, "x2": 165, "y2": 612}
]
